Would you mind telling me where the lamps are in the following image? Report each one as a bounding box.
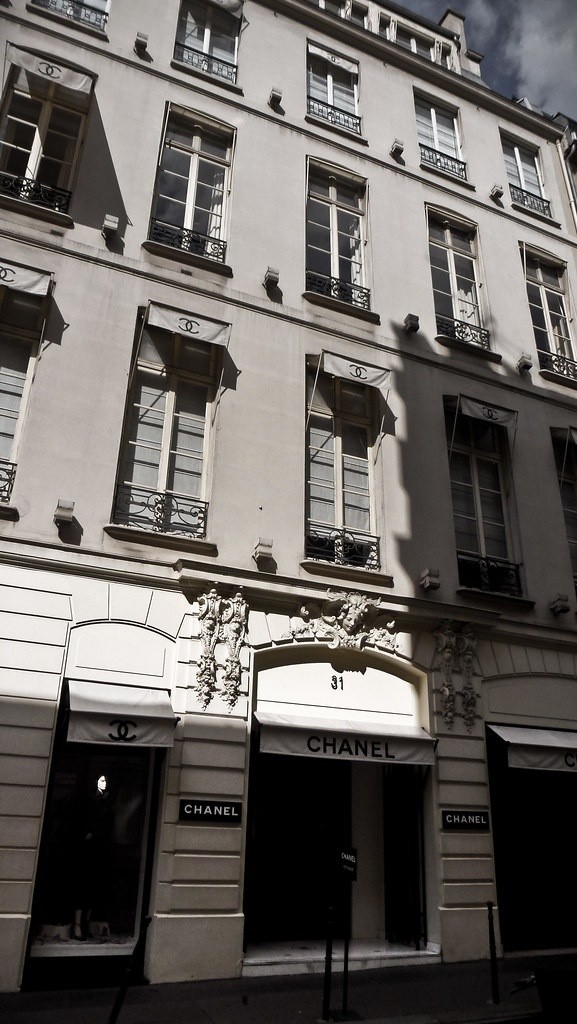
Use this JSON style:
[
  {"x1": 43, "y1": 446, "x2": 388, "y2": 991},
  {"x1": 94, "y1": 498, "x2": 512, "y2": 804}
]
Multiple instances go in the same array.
[
  {"x1": 252, "y1": 536, "x2": 274, "y2": 563},
  {"x1": 101, "y1": 213, "x2": 119, "y2": 232},
  {"x1": 490, "y1": 185, "x2": 504, "y2": 198},
  {"x1": 518, "y1": 354, "x2": 533, "y2": 370},
  {"x1": 53, "y1": 498, "x2": 76, "y2": 524},
  {"x1": 403, "y1": 313, "x2": 420, "y2": 333},
  {"x1": 418, "y1": 568, "x2": 441, "y2": 590},
  {"x1": 391, "y1": 140, "x2": 405, "y2": 156},
  {"x1": 268, "y1": 88, "x2": 282, "y2": 106},
  {"x1": 134, "y1": 31, "x2": 149, "y2": 52},
  {"x1": 548, "y1": 593, "x2": 570, "y2": 614},
  {"x1": 262, "y1": 265, "x2": 280, "y2": 287}
]
